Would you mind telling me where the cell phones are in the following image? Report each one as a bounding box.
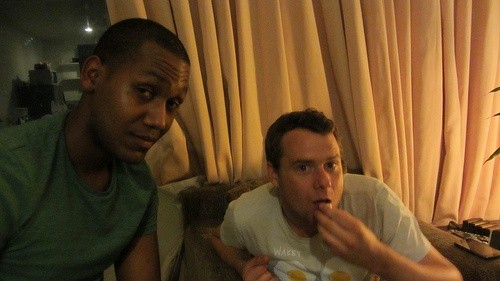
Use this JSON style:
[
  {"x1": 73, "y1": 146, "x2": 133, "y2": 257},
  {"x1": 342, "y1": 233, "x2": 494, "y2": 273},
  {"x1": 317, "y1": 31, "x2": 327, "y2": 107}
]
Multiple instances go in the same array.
[{"x1": 455, "y1": 239, "x2": 500, "y2": 260}]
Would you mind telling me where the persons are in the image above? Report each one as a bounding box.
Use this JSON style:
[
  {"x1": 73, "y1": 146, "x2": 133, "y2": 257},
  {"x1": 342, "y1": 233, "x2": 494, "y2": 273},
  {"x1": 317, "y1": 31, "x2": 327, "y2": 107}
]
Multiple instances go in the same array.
[
  {"x1": 0, "y1": 17, "x2": 191, "y2": 281},
  {"x1": 209, "y1": 105, "x2": 464, "y2": 281}
]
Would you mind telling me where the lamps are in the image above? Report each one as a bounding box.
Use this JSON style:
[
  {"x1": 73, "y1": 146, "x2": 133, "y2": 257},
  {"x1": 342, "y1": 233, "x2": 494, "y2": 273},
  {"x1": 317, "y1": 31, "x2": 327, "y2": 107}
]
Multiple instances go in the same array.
[{"x1": 85, "y1": 16, "x2": 93, "y2": 32}]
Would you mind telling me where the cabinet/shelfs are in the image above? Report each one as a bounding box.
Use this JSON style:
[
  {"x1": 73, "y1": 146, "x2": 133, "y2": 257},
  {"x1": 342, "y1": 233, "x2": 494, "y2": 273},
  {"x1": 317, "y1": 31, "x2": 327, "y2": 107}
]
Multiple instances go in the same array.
[
  {"x1": 24, "y1": 79, "x2": 67, "y2": 116},
  {"x1": 62, "y1": 61, "x2": 81, "y2": 80}
]
